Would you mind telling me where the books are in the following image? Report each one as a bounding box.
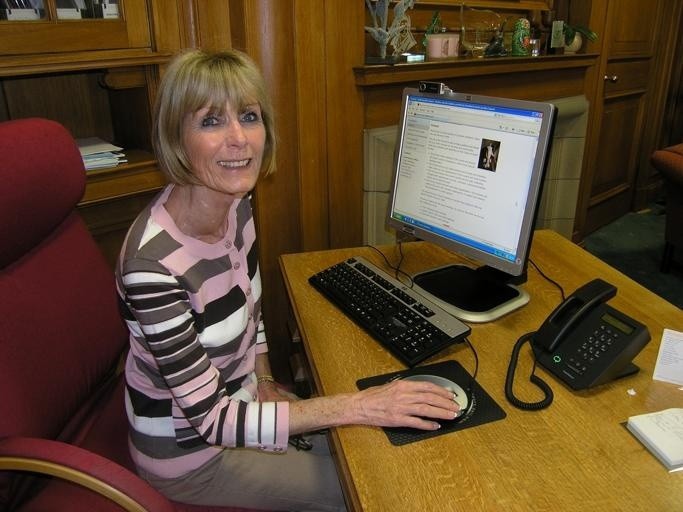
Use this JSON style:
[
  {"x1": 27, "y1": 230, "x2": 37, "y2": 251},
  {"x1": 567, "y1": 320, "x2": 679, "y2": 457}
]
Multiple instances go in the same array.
[
  {"x1": 72, "y1": 136, "x2": 129, "y2": 168},
  {"x1": 619, "y1": 407, "x2": 683, "y2": 473}
]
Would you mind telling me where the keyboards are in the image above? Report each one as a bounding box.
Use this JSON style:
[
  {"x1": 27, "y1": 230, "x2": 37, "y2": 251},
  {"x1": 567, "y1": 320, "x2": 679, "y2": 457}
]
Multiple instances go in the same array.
[{"x1": 307, "y1": 254, "x2": 471, "y2": 367}]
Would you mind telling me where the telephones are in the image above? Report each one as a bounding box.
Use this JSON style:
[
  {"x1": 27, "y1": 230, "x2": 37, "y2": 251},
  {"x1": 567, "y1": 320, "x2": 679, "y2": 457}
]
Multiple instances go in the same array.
[{"x1": 527, "y1": 279, "x2": 651, "y2": 393}]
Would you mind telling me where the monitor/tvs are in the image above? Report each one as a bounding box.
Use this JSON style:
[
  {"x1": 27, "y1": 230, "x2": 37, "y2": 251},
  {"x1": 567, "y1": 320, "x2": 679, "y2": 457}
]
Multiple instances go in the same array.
[{"x1": 384, "y1": 83, "x2": 558, "y2": 325}]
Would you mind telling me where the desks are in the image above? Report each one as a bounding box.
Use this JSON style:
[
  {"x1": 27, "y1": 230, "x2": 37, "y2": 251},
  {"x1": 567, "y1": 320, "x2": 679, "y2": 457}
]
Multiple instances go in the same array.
[{"x1": 279, "y1": 228, "x2": 683, "y2": 512}]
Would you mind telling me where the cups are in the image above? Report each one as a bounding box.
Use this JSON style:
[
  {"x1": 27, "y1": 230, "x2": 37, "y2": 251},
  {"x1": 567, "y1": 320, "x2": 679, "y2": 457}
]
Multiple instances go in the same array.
[{"x1": 529, "y1": 39, "x2": 541, "y2": 56}]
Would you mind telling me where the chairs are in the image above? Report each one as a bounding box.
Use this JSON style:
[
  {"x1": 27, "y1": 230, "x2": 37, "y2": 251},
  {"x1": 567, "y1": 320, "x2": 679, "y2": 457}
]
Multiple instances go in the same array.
[{"x1": 0, "y1": 118, "x2": 290, "y2": 512}]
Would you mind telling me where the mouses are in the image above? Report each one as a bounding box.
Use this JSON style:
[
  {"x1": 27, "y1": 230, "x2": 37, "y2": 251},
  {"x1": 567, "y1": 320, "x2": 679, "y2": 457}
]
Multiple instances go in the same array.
[{"x1": 397, "y1": 374, "x2": 471, "y2": 418}]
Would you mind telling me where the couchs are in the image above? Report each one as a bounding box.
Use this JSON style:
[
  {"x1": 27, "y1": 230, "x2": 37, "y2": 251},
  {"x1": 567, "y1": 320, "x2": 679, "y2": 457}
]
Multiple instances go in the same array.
[{"x1": 650, "y1": 142, "x2": 683, "y2": 277}]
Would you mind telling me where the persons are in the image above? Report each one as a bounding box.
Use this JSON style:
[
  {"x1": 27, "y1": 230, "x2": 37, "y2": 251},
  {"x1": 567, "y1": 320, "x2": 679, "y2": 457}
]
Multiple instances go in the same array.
[{"x1": 113, "y1": 45, "x2": 460, "y2": 511}]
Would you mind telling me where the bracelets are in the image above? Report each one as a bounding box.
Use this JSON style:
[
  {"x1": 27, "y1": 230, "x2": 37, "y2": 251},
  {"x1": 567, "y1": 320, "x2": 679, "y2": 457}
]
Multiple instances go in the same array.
[{"x1": 256, "y1": 375, "x2": 274, "y2": 383}]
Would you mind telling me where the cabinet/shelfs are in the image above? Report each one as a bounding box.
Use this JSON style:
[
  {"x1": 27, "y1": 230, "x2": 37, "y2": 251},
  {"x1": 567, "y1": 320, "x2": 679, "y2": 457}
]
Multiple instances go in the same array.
[{"x1": 0, "y1": 0, "x2": 178, "y2": 208}]
